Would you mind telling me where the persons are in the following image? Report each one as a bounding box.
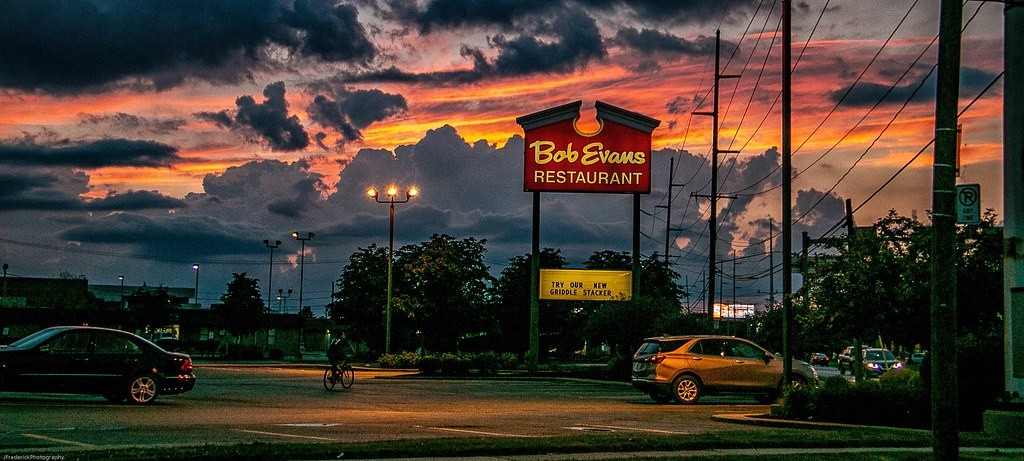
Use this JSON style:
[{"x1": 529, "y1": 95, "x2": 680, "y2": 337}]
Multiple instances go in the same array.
[{"x1": 326, "y1": 331, "x2": 355, "y2": 382}]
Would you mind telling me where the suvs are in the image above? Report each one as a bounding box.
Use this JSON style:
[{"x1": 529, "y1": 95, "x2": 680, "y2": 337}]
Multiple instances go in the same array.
[{"x1": 629, "y1": 333, "x2": 819, "y2": 405}]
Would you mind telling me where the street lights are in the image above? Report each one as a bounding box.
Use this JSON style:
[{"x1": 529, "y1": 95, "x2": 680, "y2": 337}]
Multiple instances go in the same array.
[
  {"x1": 371, "y1": 185, "x2": 418, "y2": 355},
  {"x1": 192, "y1": 263, "x2": 201, "y2": 309},
  {"x1": 262, "y1": 239, "x2": 282, "y2": 312},
  {"x1": 291, "y1": 230, "x2": 314, "y2": 311},
  {"x1": 277, "y1": 287, "x2": 292, "y2": 315},
  {"x1": 118, "y1": 275, "x2": 124, "y2": 304}
]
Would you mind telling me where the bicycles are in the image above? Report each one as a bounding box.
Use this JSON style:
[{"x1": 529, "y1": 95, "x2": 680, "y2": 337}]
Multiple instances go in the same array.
[{"x1": 324, "y1": 351, "x2": 357, "y2": 391}]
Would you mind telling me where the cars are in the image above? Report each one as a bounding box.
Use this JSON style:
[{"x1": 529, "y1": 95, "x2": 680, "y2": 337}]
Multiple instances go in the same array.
[
  {"x1": 810, "y1": 353, "x2": 830, "y2": 367},
  {"x1": 839, "y1": 345, "x2": 869, "y2": 376},
  {"x1": 0, "y1": 324, "x2": 196, "y2": 405},
  {"x1": 851, "y1": 349, "x2": 905, "y2": 380}
]
